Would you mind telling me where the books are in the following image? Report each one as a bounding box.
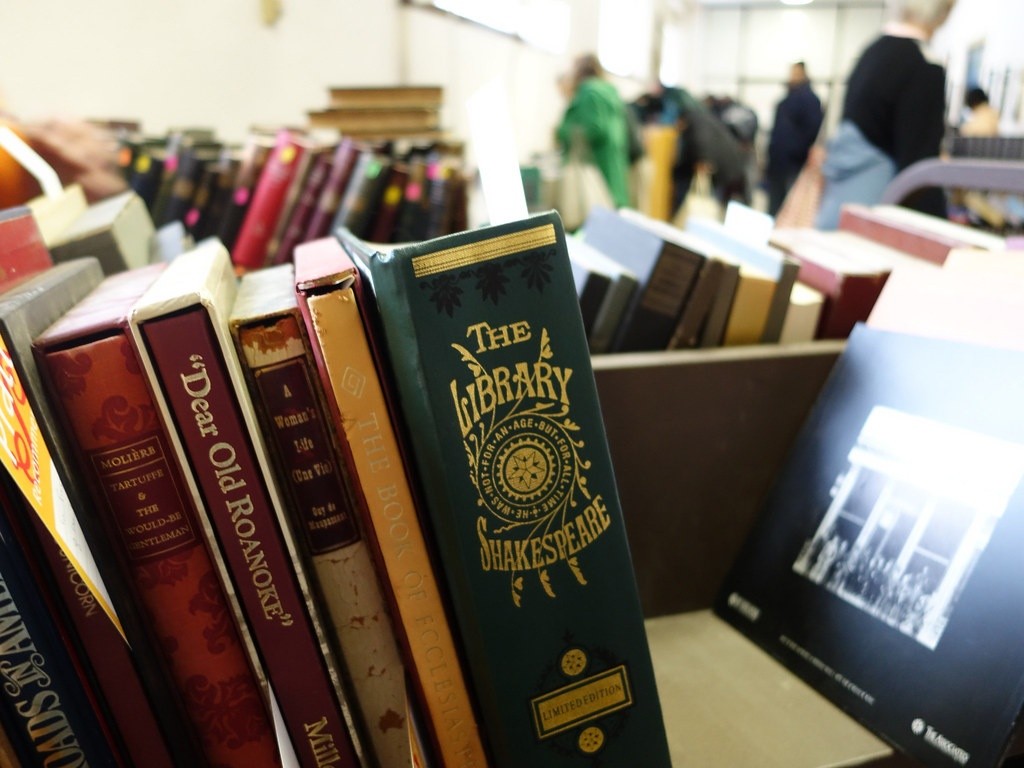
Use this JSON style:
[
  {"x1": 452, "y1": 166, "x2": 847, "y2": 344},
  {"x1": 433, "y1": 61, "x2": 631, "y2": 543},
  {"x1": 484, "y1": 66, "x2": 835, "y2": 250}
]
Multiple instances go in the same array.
[{"x1": 0, "y1": 86, "x2": 1024, "y2": 768}]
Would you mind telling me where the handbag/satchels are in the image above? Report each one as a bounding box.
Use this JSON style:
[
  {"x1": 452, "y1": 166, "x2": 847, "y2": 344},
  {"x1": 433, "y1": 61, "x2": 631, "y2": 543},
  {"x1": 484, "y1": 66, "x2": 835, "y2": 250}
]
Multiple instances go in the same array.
[{"x1": 536, "y1": 134, "x2": 613, "y2": 228}]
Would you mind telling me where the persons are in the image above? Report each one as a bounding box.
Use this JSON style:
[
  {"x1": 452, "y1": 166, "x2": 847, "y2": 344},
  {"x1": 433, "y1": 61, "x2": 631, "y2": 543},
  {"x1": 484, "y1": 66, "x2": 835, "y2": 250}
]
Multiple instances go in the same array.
[{"x1": 552, "y1": 1, "x2": 1002, "y2": 231}]
